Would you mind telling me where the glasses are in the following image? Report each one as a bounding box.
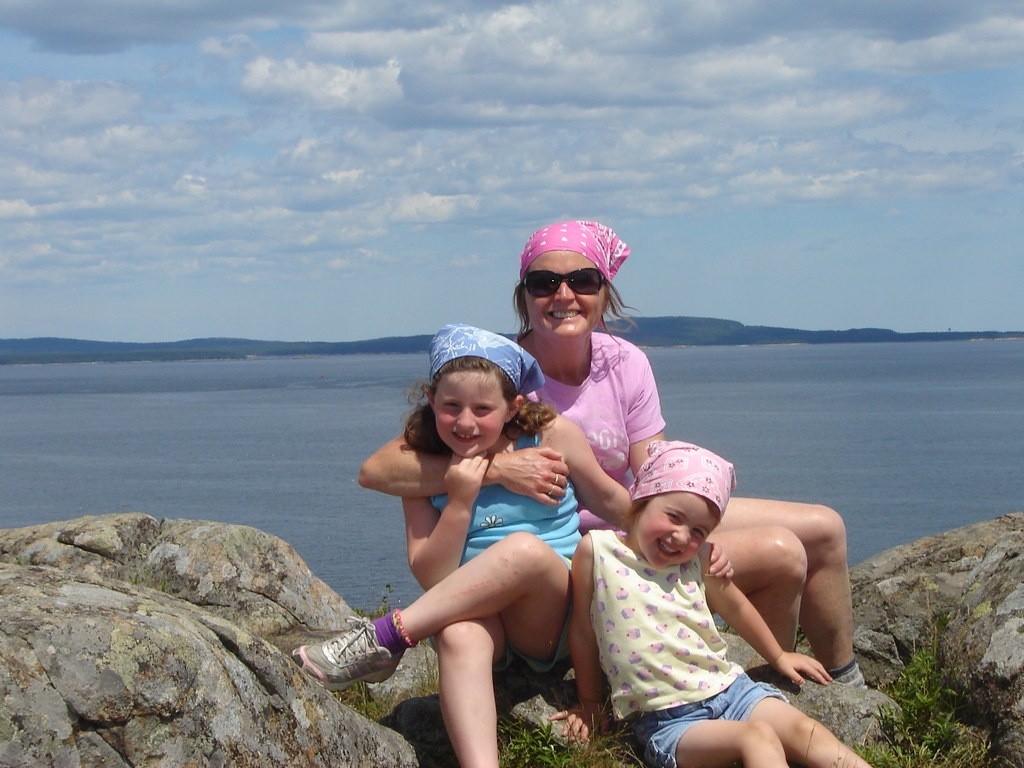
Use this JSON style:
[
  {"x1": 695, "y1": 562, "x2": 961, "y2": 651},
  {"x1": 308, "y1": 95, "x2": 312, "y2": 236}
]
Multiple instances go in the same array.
[{"x1": 523, "y1": 268, "x2": 606, "y2": 298}]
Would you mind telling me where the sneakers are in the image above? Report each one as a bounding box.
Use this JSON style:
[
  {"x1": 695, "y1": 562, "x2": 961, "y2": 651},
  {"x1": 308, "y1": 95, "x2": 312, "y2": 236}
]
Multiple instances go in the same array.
[{"x1": 292, "y1": 617, "x2": 407, "y2": 692}]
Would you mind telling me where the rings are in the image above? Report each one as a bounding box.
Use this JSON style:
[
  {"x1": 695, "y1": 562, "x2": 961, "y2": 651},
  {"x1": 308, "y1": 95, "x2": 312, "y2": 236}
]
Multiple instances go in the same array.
[
  {"x1": 548, "y1": 485, "x2": 555, "y2": 495},
  {"x1": 554, "y1": 473, "x2": 558, "y2": 484}
]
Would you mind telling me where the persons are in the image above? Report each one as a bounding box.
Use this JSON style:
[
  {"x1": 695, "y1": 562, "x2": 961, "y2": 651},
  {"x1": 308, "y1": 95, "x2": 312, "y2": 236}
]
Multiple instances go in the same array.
[
  {"x1": 358, "y1": 220, "x2": 869, "y2": 690},
  {"x1": 291, "y1": 323, "x2": 733, "y2": 768},
  {"x1": 547, "y1": 439, "x2": 875, "y2": 768}
]
